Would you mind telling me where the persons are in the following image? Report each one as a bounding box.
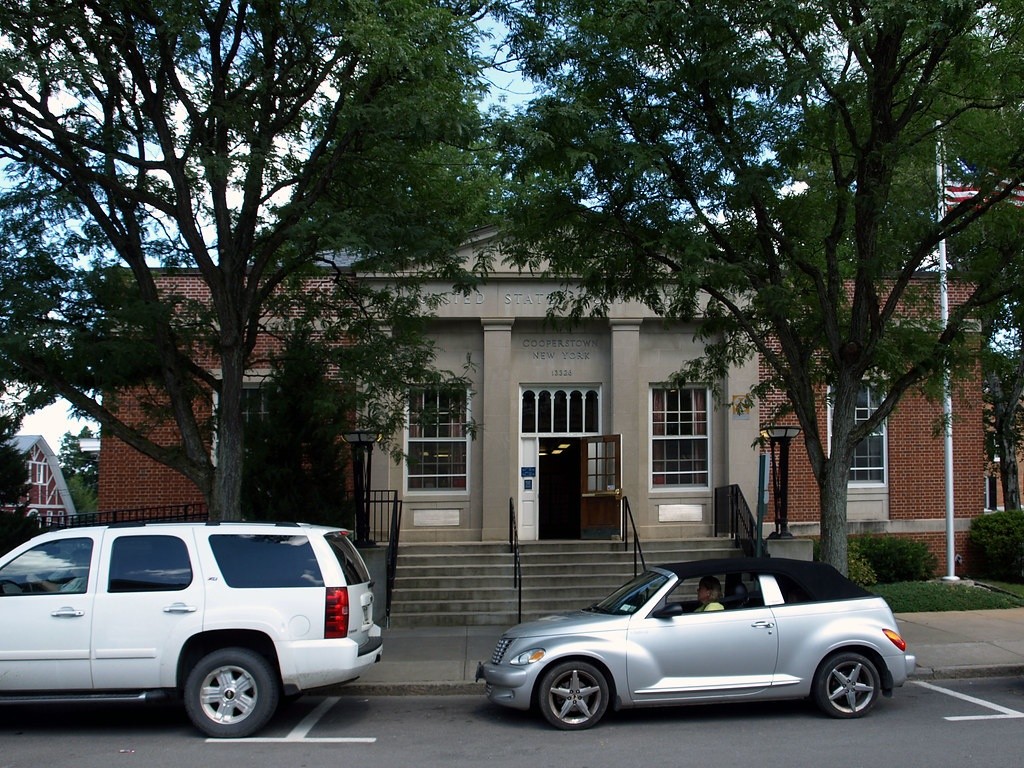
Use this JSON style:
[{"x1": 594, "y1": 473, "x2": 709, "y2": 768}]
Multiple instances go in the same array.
[
  {"x1": 24, "y1": 549, "x2": 90, "y2": 592},
  {"x1": 693, "y1": 576, "x2": 725, "y2": 612}
]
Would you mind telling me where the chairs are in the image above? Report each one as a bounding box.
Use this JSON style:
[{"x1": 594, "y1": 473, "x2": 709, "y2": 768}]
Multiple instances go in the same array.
[{"x1": 729, "y1": 581, "x2": 748, "y2": 611}]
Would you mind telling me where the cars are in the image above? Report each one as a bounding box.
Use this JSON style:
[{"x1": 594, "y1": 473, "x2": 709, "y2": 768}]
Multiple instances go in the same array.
[
  {"x1": 475, "y1": 558, "x2": 916, "y2": 732},
  {"x1": 0, "y1": 521, "x2": 384, "y2": 738}
]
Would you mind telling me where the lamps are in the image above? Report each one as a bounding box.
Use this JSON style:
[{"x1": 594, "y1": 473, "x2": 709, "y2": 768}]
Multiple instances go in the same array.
[
  {"x1": 340, "y1": 431, "x2": 382, "y2": 444},
  {"x1": 759, "y1": 427, "x2": 802, "y2": 442}
]
